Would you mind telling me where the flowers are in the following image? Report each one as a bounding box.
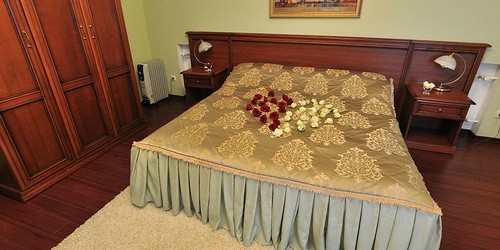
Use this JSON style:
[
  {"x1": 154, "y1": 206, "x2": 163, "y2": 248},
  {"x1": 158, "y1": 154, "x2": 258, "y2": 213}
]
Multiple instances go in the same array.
[{"x1": 245, "y1": 90, "x2": 341, "y2": 138}]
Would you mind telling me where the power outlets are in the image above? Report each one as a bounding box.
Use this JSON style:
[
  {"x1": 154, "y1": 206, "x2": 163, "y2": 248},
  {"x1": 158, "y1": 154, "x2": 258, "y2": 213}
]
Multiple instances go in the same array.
[
  {"x1": 494, "y1": 110, "x2": 500, "y2": 119},
  {"x1": 171, "y1": 75, "x2": 177, "y2": 81}
]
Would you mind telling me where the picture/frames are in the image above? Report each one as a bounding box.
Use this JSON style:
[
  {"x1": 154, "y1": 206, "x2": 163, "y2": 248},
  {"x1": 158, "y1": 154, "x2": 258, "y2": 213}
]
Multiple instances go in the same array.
[{"x1": 268, "y1": 0, "x2": 363, "y2": 19}]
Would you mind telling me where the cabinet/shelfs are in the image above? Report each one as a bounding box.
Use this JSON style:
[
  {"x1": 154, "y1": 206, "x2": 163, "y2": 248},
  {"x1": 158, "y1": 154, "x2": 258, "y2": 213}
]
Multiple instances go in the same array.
[{"x1": 0, "y1": 0, "x2": 149, "y2": 199}]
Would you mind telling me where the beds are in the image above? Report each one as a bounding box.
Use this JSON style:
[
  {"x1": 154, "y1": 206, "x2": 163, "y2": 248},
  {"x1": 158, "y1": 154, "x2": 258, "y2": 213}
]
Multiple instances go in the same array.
[{"x1": 129, "y1": 62, "x2": 442, "y2": 250}]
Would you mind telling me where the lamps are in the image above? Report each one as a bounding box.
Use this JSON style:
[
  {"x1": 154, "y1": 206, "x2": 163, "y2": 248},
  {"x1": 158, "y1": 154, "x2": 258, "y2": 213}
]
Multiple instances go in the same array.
[
  {"x1": 434, "y1": 52, "x2": 467, "y2": 92},
  {"x1": 193, "y1": 39, "x2": 213, "y2": 69}
]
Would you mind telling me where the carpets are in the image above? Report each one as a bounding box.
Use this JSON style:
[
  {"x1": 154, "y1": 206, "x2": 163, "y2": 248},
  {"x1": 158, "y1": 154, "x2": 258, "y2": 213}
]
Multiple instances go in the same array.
[{"x1": 51, "y1": 186, "x2": 292, "y2": 250}]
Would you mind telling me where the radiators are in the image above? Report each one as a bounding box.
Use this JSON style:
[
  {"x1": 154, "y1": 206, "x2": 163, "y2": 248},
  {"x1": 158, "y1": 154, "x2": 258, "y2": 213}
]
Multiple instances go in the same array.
[{"x1": 137, "y1": 59, "x2": 169, "y2": 106}]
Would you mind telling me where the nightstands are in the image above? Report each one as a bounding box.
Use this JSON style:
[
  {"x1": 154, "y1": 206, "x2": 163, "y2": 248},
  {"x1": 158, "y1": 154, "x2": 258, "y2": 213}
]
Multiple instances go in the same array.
[
  {"x1": 180, "y1": 66, "x2": 228, "y2": 109},
  {"x1": 400, "y1": 83, "x2": 476, "y2": 155}
]
